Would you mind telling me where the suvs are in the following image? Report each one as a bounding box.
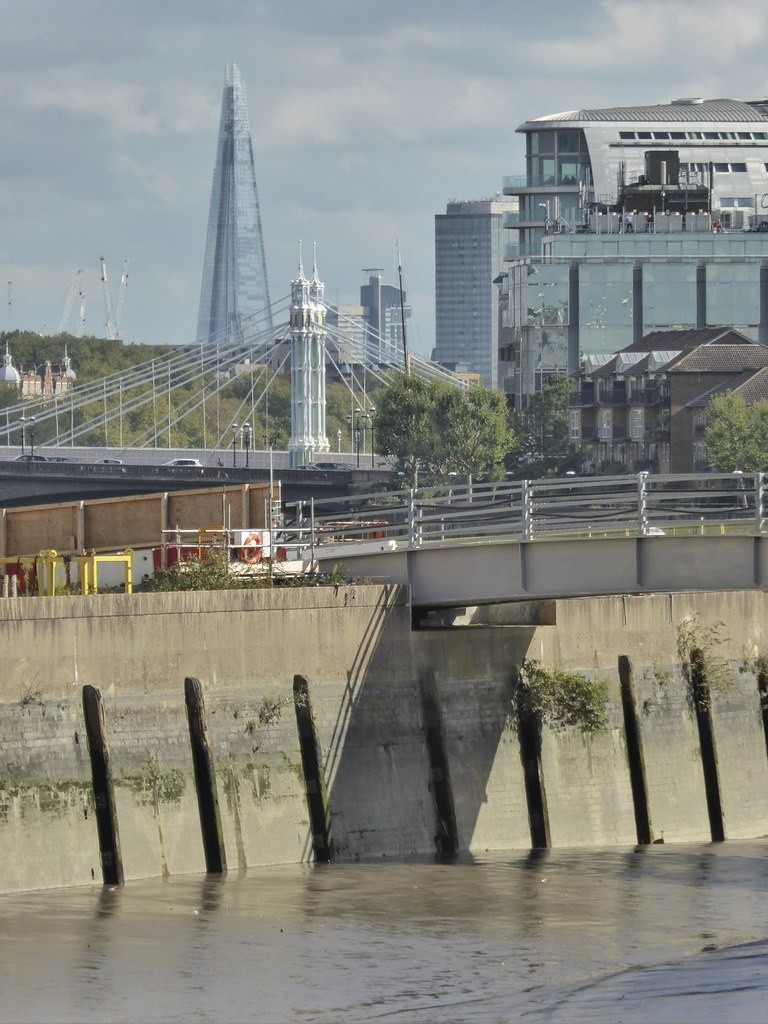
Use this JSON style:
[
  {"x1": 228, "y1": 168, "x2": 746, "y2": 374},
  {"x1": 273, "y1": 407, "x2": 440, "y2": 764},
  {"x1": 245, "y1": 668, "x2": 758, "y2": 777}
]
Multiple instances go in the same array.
[{"x1": 161, "y1": 458, "x2": 203, "y2": 467}]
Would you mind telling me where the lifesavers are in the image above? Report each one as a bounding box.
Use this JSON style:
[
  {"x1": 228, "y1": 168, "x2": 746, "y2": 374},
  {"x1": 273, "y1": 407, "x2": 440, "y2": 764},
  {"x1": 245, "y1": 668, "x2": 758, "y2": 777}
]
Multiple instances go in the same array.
[{"x1": 241, "y1": 535, "x2": 263, "y2": 564}]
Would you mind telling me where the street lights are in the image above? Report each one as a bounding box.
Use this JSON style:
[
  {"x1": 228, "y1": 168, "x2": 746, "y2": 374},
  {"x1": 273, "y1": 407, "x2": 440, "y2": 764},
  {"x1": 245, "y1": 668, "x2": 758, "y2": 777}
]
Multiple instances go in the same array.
[
  {"x1": 231, "y1": 422, "x2": 250, "y2": 468},
  {"x1": 346, "y1": 406, "x2": 376, "y2": 468},
  {"x1": 19, "y1": 417, "x2": 36, "y2": 455}
]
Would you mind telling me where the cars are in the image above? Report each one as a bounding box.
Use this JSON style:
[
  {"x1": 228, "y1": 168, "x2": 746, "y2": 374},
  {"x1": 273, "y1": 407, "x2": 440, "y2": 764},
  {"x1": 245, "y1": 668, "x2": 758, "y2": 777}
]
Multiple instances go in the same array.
[
  {"x1": 93, "y1": 459, "x2": 123, "y2": 464},
  {"x1": 11, "y1": 453, "x2": 72, "y2": 462}
]
